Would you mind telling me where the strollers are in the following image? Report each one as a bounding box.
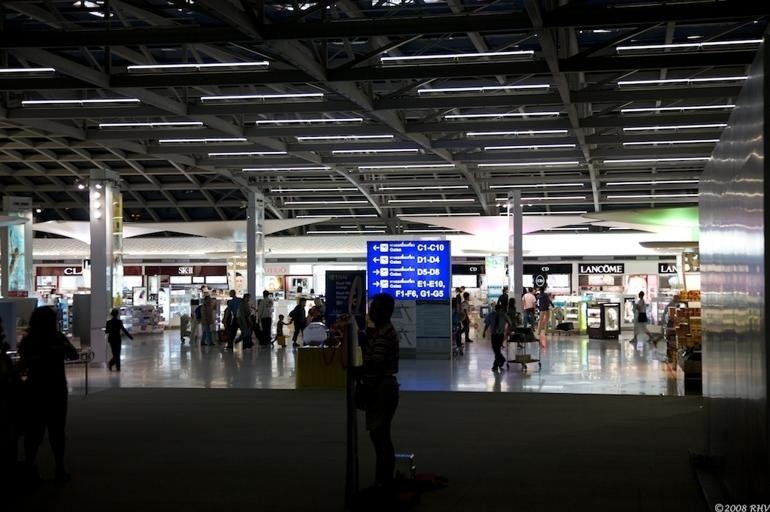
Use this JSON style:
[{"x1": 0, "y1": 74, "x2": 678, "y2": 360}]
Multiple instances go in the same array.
[
  {"x1": 176, "y1": 312, "x2": 191, "y2": 343},
  {"x1": 452, "y1": 325, "x2": 465, "y2": 357}
]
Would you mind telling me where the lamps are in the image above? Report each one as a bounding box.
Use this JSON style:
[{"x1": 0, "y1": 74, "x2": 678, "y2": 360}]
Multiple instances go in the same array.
[
  {"x1": 36, "y1": 207, "x2": 42, "y2": 214},
  {"x1": 99, "y1": 121, "x2": 208, "y2": 130},
  {"x1": 92, "y1": 183, "x2": 102, "y2": 219},
  {"x1": 127, "y1": 61, "x2": 271, "y2": 75},
  {"x1": 476, "y1": 161, "x2": 590, "y2": 232},
  {"x1": 2, "y1": 67, "x2": 57, "y2": 81},
  {"x1": 159, "y1": 138, "x2": 248, "y2": 147},
  {"x1": 358, "y1": 164, "x2": 479, "y2": 219},
  {"x1": 602, "y1": 157, "x2": 709, "y2": 203},
  {"x1": 381, "y1": 51, "x2": 577, "y2": 152},
  {"x1": 615, "y1": 41, "x2": 763, "y2": 152},
  {"x1": 240, "y1": 168, "x2": 385, "y2": 234},
  {"x1": 78, "y1": 182, "x2": 87, "y2": 191},
  {"x1": 21, "y1": 98, "x2": 140, "y2": 110},
  {"x1": 200, "y1": 92, "x2": 425, "y2": 161}
]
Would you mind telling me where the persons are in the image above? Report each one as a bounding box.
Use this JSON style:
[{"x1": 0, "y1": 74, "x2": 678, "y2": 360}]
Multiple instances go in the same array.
[
  {"x1": 15, "y1": 305, "x2": 79, "y2": 484},
  {"x1": 104, "y1": 308, "x2": 133, "y2": 372},
  {"x1": 198, "y1": 283, "x2": 656, "y2": 371},
  {"x1": 352, "y1": 295, "x2": 400, "y2": 486}
]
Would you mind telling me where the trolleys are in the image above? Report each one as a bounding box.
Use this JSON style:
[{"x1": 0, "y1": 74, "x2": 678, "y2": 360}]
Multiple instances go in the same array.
[
  {"x1": 549, "y1": 306, "x2": 575, "y2": 337},
  {"x1": 505, "y1": 330, "x2": 542, "y2": 372}
]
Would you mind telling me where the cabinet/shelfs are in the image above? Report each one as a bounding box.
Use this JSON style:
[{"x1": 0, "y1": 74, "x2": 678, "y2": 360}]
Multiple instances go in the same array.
[{"x1": 585, "y1": 303, "x2": 621, "y2": 341}]
